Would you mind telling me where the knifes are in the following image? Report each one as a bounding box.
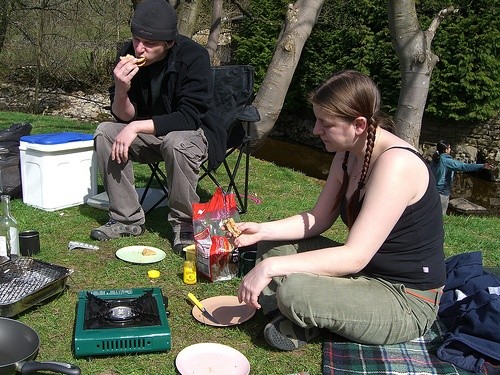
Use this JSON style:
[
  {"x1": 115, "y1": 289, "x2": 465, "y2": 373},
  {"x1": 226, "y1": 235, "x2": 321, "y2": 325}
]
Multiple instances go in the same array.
[{"x1": 186, "y1": 291, "x2": 222, "y2": 324}]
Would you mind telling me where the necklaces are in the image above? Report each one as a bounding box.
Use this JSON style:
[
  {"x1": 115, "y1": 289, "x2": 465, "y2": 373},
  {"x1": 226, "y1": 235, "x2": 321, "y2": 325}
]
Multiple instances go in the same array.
[{"x1": 353, "y1": 160, "x2": 363, "y2": 180}]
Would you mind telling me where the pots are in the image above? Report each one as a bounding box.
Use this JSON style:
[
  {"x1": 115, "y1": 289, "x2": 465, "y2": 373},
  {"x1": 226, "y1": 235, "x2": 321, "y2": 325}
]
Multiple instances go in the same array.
[{"x1": 0, "y1": 316, "x2": 81, "y2": 375}]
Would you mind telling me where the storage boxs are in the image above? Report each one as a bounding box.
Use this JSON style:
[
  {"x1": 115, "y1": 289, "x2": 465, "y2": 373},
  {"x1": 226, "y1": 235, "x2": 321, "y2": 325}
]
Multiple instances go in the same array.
[
  {"x1": 18, "y1": 132, "x2": 98, "y2": 212},
  {"x1": 182, "y1": 244, "x2": 196, "y2": 262}
]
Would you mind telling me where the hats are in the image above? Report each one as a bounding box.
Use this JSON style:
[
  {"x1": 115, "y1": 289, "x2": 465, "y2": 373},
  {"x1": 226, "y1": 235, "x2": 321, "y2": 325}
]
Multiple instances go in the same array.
[{"x1": 131, "y1": 0, "x2": 178, "y2": 40}]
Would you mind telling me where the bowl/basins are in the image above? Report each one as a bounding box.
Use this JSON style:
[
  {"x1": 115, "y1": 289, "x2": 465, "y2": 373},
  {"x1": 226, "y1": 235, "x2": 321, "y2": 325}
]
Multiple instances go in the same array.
[{"x1": 182, "y1": 245, "x2": 197, "y2": 262}]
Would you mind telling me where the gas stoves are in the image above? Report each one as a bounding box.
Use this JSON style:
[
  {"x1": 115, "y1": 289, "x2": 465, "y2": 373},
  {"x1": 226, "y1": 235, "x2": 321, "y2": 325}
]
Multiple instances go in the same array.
[{"x1": 73, "y1": 287, "x2": 171, "y2": 356}]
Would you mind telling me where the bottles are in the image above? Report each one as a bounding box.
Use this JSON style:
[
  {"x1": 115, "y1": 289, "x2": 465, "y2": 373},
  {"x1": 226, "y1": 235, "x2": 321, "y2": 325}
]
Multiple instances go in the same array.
[
  {"x1": 242, "y1": 251, "x2": 258, "y2": 275},
  {"x1": 182, "y1": 261, "x2": 197, "y2": 284},
  {"x1": 0, "y1": 195, "x2": 20, "y2": 256}
]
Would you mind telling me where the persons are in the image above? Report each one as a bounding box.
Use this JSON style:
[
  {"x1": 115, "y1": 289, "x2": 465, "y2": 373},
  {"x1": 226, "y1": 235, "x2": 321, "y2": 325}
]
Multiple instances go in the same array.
[
  {"x1": 216, "y1": 69, "x2": 447, "y2": 350},
  {"x1": 426, "y1": 139, "x2": 492, "y2": 215},
  {"x1": 90, "y1": 0, "x2": 212, "y2": 251}
]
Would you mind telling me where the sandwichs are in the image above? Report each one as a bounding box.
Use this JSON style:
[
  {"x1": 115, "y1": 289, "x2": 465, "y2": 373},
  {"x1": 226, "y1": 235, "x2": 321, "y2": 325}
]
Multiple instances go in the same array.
[
  {"x1": 119, "y1": 54, "x2": 146, "y2": 67},
  {"x1": 142, "y1": 248, "x2": 156, "y2": 256},
  {"x1": 225, "y1": 217, "x2": 242, "y2": 237}
]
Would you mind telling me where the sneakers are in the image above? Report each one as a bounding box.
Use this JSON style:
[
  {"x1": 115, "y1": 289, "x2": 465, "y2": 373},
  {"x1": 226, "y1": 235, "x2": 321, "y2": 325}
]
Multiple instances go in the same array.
[
  {"x1": 172, "y1": 230, "x2": 196, "y2": 252},
  {"x1": 90, "y1": 217, "x2": 145, "y2": 240}
]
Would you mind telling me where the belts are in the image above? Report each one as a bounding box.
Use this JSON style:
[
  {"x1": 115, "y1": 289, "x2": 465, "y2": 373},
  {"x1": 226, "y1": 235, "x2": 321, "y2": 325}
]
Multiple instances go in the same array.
[{"x1": 405, "y1": 286, "x2": 443, "y2": 304}]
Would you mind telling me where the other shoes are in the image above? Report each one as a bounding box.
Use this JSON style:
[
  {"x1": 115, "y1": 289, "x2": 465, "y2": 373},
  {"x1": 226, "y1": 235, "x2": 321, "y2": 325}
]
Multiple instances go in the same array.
[{"x1": 263, "y1": 310, "x2": 325, "y2": 352}]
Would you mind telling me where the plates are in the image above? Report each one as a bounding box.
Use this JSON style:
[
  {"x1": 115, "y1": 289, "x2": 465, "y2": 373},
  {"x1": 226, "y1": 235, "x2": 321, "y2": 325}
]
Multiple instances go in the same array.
[
  {"x1": 116, "y1": 246, "x2": 166, "y2": 264},
  {"x1": 175, "y1": 341, "x2": 250, "y2": 375},
  {"x1": 192, "y1": 295, "x2": 258, "y2": 326}
]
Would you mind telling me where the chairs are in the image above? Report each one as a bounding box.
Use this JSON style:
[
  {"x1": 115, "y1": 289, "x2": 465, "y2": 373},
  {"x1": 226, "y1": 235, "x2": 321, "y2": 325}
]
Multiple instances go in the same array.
[{"x1": 139, "y1": 63, "x2": 261, "y2": 215}]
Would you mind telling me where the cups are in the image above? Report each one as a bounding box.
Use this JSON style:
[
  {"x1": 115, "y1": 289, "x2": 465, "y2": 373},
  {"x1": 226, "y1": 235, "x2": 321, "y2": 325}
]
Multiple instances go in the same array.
[{"x1": 19, "y1": 230, "x2": 41, "y2": 256}]
